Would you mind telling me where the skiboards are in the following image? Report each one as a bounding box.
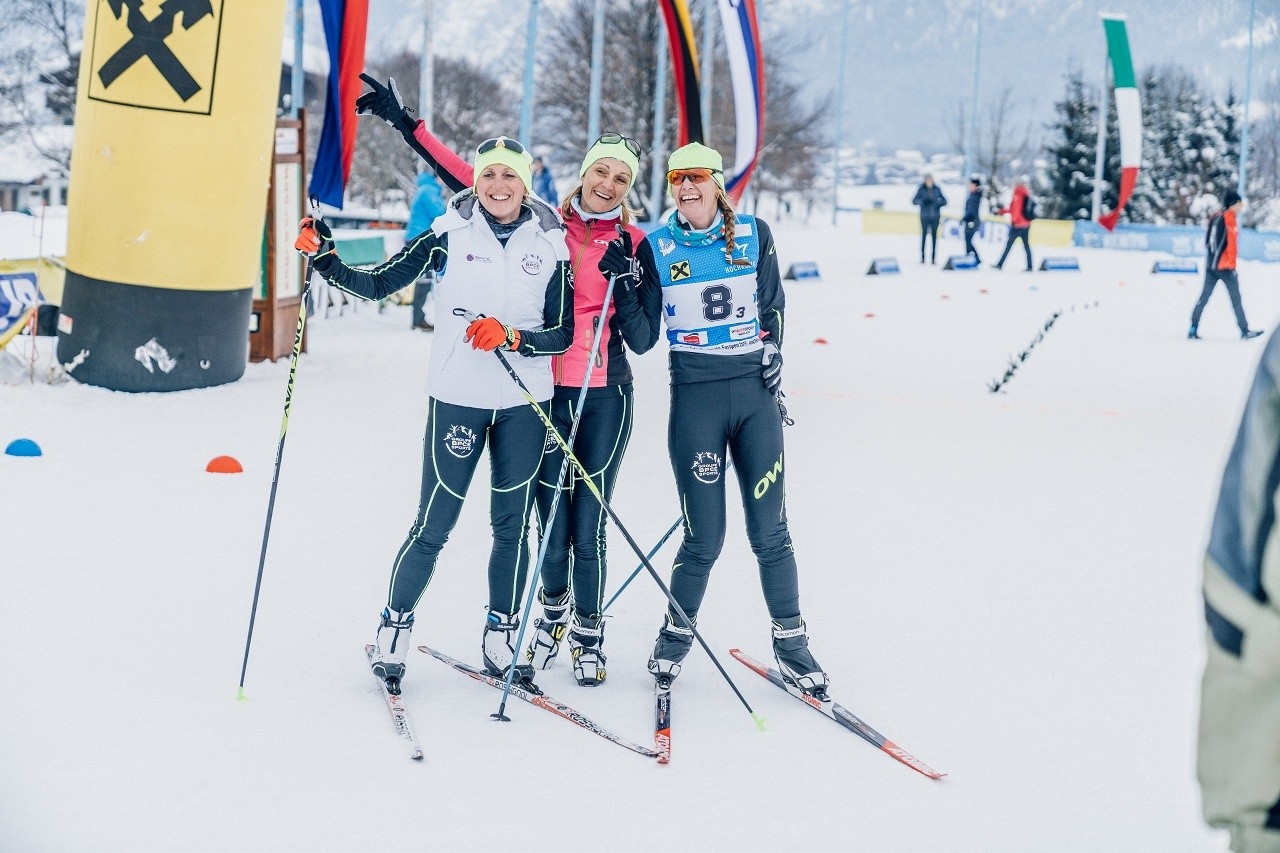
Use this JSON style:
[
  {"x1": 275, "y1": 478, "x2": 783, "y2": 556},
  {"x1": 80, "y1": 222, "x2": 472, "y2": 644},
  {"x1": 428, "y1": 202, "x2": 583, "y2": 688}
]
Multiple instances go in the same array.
[
  {"x1": 653, "y1": 647, "x2": 950, "y2": 781},
  {"x1": 364, "y1": 642, "x2": 665, "y2": 761}
]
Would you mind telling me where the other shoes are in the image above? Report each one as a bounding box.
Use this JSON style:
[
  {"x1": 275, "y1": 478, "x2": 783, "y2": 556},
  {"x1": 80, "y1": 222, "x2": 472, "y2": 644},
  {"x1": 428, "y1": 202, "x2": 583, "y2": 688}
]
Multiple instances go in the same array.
[
  {"x1": 1188, "y1": 331, "x2": 1198, "y2": 338},
  {"x1": 412, "y1": 318, "x2": 434, "y2": 332},
  {"x1": 1244, "y1": 329, "x2": 1263, "y2": 339}
]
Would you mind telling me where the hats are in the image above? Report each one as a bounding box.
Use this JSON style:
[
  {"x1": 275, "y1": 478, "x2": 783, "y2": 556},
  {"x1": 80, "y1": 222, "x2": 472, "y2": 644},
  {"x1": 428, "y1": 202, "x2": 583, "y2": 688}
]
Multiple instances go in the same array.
[
  {"x1": 579, "y1": 134, "x2": 639, "y2": 197},
  {"x1": 473, "y1": 133, "x2": 534, "y2": 198},
  {"x1": 667, "y1": 143, "x2": 725, "y2": 200},
  {"x1": 1224, "y1": 190, "x2": 1241, "y2": 209}
]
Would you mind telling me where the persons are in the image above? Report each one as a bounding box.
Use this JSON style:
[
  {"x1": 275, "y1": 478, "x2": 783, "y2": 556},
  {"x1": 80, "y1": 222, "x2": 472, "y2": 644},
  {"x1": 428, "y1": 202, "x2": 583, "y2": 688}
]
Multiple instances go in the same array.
[
  {"x1": 1197, "y1": 326, "x2": 1280, "y2": 853},
  {"x1": 296, "y1": 135, "x2": 575, "y2": 685},
  {"x1": 960, "y1": 179, "x2": 981, "y2": 265},
  {"x1": 354, "y1": 73, "x2": 647, "y2": 687},
  {"x1": 913, "y1": 176, "x2": 947, "y2": 264},
  {"x1": 992, "y1": 179, "x2": 1033, "y2": 271},
  {"x1": 531, "y1": 156, "x2": 559, "y2": 208},
  {"x1": 598, "y1": 141, "x2": 831, "y2": 697},
  {"x1": 1187, "y1": 191, "x2": 1265, "y2": 340},
  {"x1": 403, "y1": 170, "x2": 447, "y2": 329}
]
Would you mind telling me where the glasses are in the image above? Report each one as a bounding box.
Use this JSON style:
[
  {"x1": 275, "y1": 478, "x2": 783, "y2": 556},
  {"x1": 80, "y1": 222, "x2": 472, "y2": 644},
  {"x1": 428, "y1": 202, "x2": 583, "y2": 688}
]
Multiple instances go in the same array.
[
  {"x1": 476, "y1": 138, "x2": 533, "y2": 173},
  {"x1": 666, "y1": 167, "x2": 723, "y2": 186},
  {"x1": 589, "y1": 132, "x2": 642, "y2": 163}
]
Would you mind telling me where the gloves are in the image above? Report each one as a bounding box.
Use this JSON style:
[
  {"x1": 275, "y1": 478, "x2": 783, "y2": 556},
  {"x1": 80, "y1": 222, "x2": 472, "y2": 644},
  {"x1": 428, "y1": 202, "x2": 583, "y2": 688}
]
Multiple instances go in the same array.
[
  {"x1": 597, "y1": 230, "x2": 632, "y2": 282},
  {"x1": 761, "y1": 343, "x2": 782, "y2": 395},
  {"x1": 463, "y1": 318, "x2": 521, "y2": 352},
  {"x1": 354, "y1": 73, "x2": 410, "y2": 128},
  {"x1": 294, "y1": 217, "x2": 336, "y2": 270}
]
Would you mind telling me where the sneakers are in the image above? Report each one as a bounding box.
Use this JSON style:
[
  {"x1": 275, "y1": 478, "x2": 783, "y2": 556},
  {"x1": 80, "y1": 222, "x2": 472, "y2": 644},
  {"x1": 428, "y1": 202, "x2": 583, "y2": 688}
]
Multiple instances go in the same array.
[
  {"x1": 482, "y1": 608, "x2": 535, "y2": 683},
  {"x1": 647, "y1": 611, "x2": 697, "y2": 681},
  {"x1": 773, "y1": 615, "x2": 828, "y2": 695},
  {"x1": 527, "y1": 586, "x2": 573, "y2": 672},
  {"x1": 371, "y1": 604, "x2": 414, "y2": 681},
  {"x1": 569, "y1": 611, "x2": 605, "y2": 686}
]
